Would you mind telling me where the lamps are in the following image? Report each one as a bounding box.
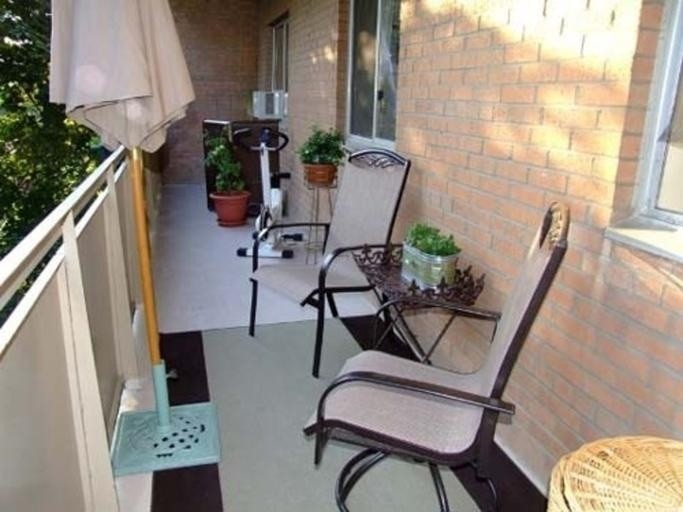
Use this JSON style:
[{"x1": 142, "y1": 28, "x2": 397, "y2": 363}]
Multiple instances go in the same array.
[{"x1": 298, "y1": 126, "x2": 348, "y2": 188}]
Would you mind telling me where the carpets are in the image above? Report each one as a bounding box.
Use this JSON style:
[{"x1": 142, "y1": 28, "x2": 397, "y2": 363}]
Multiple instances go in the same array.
[{"x1": 150, "y1": 314, "x2": 548, "y2": 512}]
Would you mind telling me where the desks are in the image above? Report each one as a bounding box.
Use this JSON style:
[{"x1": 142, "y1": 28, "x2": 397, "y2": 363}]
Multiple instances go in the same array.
[{"x1": 350, "y1": 240, "x2": 486, "y2": 365}]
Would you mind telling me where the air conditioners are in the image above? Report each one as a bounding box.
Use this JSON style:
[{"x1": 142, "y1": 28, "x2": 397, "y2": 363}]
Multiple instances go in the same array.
[{"x1": 250, "y1": 91, "x2": 288, "y2": 123}]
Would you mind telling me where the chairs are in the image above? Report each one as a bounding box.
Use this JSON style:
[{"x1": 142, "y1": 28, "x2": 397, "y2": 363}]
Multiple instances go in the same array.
[
  {"x1": 250, "y1": 148, "x2": 411, "y2": 380},
  {"x1": 303, "y1": 196, "x2": 573, "y2": 511}
]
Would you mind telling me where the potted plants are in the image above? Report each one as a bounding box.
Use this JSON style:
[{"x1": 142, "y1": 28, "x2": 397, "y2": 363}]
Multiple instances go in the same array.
[
  {"x1": 204, "y1": 136, "x2": 248, "y2": 229},
  {"x1": 399, "y1": 222, "x2": 462, "y2": 294}
]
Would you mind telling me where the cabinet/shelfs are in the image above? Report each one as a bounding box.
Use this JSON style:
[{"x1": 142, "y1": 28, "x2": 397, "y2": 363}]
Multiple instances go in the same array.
[{"x1": 202, "y1": 118, "x2": 281, "y2": 216}]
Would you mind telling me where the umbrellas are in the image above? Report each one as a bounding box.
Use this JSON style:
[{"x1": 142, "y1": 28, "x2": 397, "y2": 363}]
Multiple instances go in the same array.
[{"x1": 49, "y1": 1, "x2": 197, "y2": 364}]
